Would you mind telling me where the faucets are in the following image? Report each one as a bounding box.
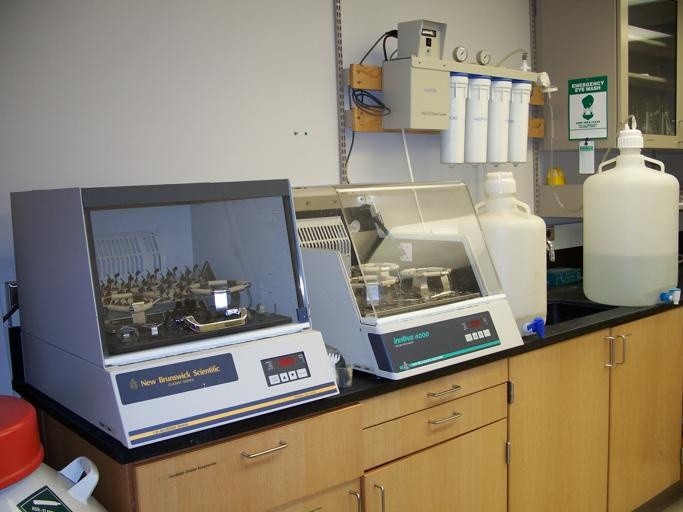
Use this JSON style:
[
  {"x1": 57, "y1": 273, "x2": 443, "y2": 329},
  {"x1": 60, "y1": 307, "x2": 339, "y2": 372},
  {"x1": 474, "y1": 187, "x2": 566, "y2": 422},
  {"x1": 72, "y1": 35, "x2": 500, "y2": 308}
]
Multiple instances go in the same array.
[
  {"x1": 523, "y1": 315, "x2": 544, "y2": 339},
  {"x1": 660, "y1": 287, "x2": 680, "y2": 306}
]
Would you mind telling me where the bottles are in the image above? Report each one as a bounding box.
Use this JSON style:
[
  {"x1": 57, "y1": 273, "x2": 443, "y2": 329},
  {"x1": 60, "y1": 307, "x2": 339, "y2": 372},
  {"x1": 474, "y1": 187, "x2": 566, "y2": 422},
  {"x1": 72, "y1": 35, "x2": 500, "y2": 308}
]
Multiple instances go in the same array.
[{"x1": 625, "y1": 101, "x2": 676, "y2": 137}]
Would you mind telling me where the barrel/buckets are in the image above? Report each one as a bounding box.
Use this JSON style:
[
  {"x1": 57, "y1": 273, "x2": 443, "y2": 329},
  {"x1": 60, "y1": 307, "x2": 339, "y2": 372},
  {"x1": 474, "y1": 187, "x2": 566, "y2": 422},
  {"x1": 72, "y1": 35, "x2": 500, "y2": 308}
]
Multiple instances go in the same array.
[{"x1": 0, "y1": 394, "x2": 114, "y2": 512}]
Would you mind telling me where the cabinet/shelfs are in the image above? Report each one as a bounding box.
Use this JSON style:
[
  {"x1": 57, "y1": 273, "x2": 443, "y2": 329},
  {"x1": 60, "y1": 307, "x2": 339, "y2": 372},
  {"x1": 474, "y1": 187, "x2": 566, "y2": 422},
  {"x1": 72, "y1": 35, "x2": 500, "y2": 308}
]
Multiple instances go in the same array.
[
  {"x1": 507, "y1": 306, "x2": 683, "y2": 512},
  {"x1": 537, "y1": 0, "x2": 682, "y2": 150},
  {"x1": 35, "y1": 357, "x2": 508, "y2": 511}
]
